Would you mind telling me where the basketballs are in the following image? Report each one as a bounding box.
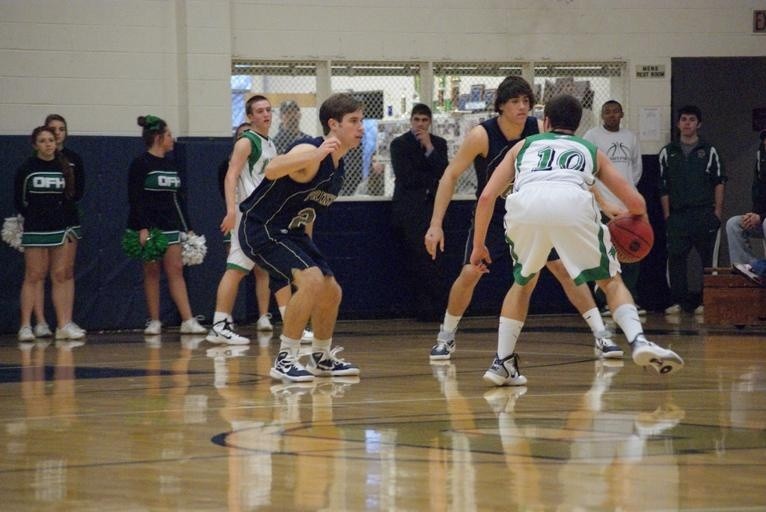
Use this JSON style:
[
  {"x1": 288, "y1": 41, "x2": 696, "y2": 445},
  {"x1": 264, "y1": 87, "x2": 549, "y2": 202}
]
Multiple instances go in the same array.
[{"x1": 607, "y1": 213, "x2": 654, "y2": 264}]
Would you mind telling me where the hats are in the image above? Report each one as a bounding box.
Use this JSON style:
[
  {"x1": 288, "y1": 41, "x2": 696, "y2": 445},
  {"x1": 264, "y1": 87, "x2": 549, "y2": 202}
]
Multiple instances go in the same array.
[{"x1": 279, "y1": 101, "x2": 299, "y2": 113}]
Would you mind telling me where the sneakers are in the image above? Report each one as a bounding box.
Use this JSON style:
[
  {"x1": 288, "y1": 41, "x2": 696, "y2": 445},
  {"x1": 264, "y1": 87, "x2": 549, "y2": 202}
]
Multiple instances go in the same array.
[
  {"x1": 34, "y1": 324, "x2": 53, "y2": 338},
  {"x1": 694, "y1": 305, "x2": 704, "y2": 314},
  {"x1": 665, "y1": 304, "x2": 680, "y2": 314},
  {"x1": 483, "y1": 352, "x2": 527, "y2": 386},
  {"x1": 55, "y1": 323, "x2": 85, "y2": 339},
  {"x1": 593, "y1": 332, "x2": 624, "y2": 358},
  {"x1": 635, "y1": 304, "x2": 647, "y2": 315},
  {"x1": 306, "y1": 345, "x2": 360, "y2": 376},
  {"x1": 300, "y1": 329, "x2": 314, "y2": 344},
  {"x1": 257, "y1": 313, "x2": 275, "y2": 331},
  {"x1": 430, "y1": 324, "x2": 458, "y2": 360},
  {"x1": 627, "y1": 333, "x2": 684, "y2": 375},
  {"x1": 180, "y1": 314, "x2": 207, "y2": 333},
  {"x1": 16, "y1": 326, "x2": 36, "y2": 341},
  {"x1": 143, "y1": 320, "x2": 161, "y2": 334},
  {"x1": 600, "y1": 304, "x2": 612, "y2": 316},
  {"x1": 733, "y1": 262, "x2": 762, "y2": 285},
  {"x1": 270, "y1": 351, "x2": 315, "y2": 382},
  {"x1": 205, "y1": 319, "x2": 250, "y2": 344}
]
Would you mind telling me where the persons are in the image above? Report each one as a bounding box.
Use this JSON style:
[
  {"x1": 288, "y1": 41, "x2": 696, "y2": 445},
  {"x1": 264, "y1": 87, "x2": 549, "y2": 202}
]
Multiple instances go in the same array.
[
  {"x1": 14, "y1": 125, "x2": 86, "y2": 341},
  {"x1": 582, "y1": 100, "x2": 648, "y2": 318},
  {"x1": 33, "y1": 114, "x2": 89, "y2": 338},
  {"x1": 237, "y1": 93, "x2": 364, "y2": 383},
  {"x1": 391, "y1": 103, "x2": 449, "y2": 322},
  {"x1": 273, "y1": 101, "x2": 312, "y2": 154},
  {"x1": 658, "y1": 106, "x2": 727, "y2": 315},
  {"x1": 126, "y1": 115, "x2": 208, "y2": 336},
  {"x1": 369, "y1": 151, "x2": 384, "y2": 194},
  {"x1": 205, "y1": 95, "x2": 314, "y2": 345},
  {"x1": 724, "y1": 132, "x2": 766, "y2": 263},
  {"x1": 354, "y1": 92, "x2": 377, "y2": 176},
  {"x1": 425, "y1": 76, "x2": 625, "y2": 361},
  {"x1": 218, "y1": 123, "x2": 276, "y2": 332},
  {"x1": 470, "y1": 94, "x2": 685, "y2": 386},
  {"x1": 732, "y1": 258, "x2": 765, "y2": 285}
]
